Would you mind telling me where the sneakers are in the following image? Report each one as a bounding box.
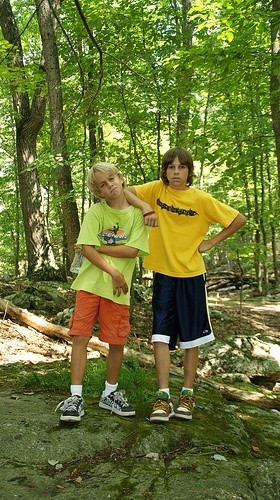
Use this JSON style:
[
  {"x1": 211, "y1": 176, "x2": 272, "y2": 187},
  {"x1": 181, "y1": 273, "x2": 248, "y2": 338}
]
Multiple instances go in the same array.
[
  {"x1": 99, "y1": 390, "x2": 135, "y2": 416},
  {"x1": 174, "y1": 389, "x2": 196, "y2": 419},
  {"x1": 149, "y1": 391, "x2": 175, "y2": 422},
  {"x1": 54, "y1": 395, "x2": 85, "y2": 422}
]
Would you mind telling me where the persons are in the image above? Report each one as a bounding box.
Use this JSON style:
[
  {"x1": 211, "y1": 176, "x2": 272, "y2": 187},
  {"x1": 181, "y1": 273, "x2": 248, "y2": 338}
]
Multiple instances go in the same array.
[
  {"x1": 123, "y1": 147, "x2": 247, "y2": 422},
  {"x1": 55, "y1": 162, "x2": 151, "y2": 423}
]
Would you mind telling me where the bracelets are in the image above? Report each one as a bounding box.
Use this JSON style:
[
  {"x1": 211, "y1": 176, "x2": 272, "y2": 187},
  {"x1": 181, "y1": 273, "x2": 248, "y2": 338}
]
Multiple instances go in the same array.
[{"x1": 143, "y1": 210, "x2": 156, "y2": 217}]
